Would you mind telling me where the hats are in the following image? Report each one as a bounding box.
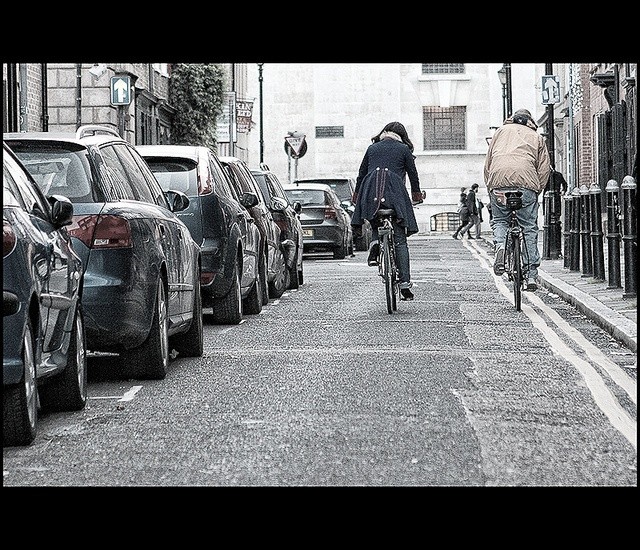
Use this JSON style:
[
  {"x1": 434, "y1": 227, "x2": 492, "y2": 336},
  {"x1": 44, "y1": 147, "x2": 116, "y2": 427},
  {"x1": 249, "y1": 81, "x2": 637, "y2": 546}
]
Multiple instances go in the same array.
[{"x1": 514, "y1": 109, "x2": 531, "y2": 117}]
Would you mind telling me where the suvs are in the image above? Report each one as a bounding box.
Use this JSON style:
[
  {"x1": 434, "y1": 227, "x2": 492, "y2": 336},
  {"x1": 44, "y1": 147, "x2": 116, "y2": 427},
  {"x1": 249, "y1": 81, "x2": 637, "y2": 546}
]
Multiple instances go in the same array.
[
  {"x1": 293, "y1": 178, "x2": 372, "y2": 250},
  {"x1": 133, "y1": 144, "x2": 262, "y2": 324},
  {"x1": 3, "y1": 141, "x2": 88, "y2": 447},
  {"x1": 3, "y1": 125, "x2": 203, "y2": 379}
]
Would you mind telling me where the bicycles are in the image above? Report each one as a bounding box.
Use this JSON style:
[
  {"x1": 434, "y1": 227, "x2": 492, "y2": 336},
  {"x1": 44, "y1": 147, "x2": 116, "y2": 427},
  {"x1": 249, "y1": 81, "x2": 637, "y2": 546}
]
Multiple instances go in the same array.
[
  {"x1": 369, "y1": 200, "x2": 423, "y2": 314},
  {"x1": 498, "y1": 193, "x2": 538, "y2": 311}
]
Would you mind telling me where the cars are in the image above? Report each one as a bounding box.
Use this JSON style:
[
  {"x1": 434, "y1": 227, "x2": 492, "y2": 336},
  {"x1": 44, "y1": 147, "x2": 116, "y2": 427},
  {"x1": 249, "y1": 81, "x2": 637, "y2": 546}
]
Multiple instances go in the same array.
[
  {"x1": 282, "y1": 183, "x2": 353, "y2": 259},
  {"x1": 249, "y1": 171, "x2": 303, "y2": 289},
  {"x1": 217, "y1": 156, "x2": 291, "y2": 305}
]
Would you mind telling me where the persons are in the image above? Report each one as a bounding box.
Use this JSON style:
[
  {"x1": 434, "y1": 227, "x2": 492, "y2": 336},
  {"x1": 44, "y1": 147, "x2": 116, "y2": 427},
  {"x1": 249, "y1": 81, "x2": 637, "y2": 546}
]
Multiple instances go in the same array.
[
  {"x1": 351, "y1": 121, "x2": 422, "y2": 299},
  {"x1": 453, "y1": 196, "x2": 474, "y2": 240},
  {"x1": 459, "y1": 183, "x2": 483, "y2": 241},
  {"x1": 461, "y1": 186, "x2": 468, "y2": 194},
  {"x1": 484, "y1": 109, "x2": 551, "y2": 291},
  {"x1": 543, "y1": 165, "x2": 568, "y2": 216}
]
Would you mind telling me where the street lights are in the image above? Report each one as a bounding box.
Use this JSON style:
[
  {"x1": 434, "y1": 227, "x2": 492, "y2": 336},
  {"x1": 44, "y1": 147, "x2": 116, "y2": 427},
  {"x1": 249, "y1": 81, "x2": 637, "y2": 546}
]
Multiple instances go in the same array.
[{"x1": 498, "y1": 68, "x2": 506, "y2": 121}]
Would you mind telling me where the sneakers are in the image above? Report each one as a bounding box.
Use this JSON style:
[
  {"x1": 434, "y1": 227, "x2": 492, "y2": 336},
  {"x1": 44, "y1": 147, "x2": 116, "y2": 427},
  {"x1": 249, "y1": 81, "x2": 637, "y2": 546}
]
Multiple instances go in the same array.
[
  {"x1": 400, "y1": 289, "x2": 413, "y2": 301},
  {"x1": 468, "y1": 235, "x2": 474, "y2": 239},
  {"x1": 527, "y1": 277, "x2": 537, "y2": 290},
  {"x1": 452, "y1": 233, "x2": 460, "y2": 239},
  {"x1": 476, "y1": 235, "x2": 481, "y2": 238},
  {"x1": 494, "y1": 245, "x2": 505, "y2": 275},
  {"x1": 368, "y1": 244, "x2": 380, "y2": 264},
  {"x1": 459, "y1": 231, "x2": 463, "y2": 238}
]
